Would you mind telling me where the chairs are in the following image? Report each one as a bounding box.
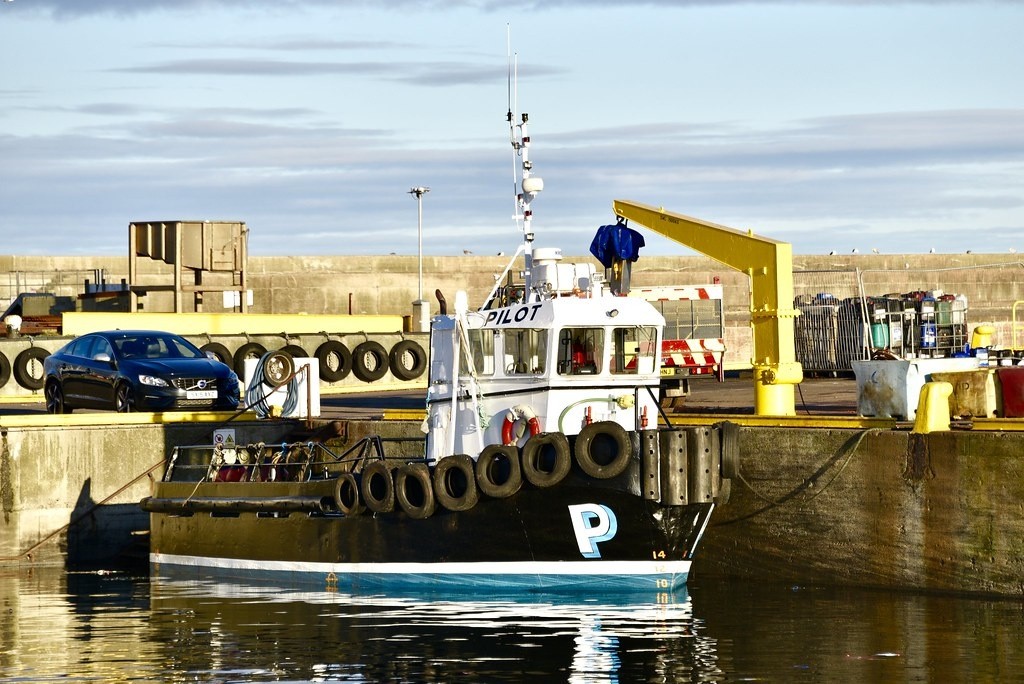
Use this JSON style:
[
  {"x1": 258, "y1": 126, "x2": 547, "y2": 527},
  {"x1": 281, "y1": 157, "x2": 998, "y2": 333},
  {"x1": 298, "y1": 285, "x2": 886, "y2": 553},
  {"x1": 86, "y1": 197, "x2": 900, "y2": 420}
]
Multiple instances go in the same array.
[{"x1": 121, "y1": 341, "x2": 135, "y2": 356}]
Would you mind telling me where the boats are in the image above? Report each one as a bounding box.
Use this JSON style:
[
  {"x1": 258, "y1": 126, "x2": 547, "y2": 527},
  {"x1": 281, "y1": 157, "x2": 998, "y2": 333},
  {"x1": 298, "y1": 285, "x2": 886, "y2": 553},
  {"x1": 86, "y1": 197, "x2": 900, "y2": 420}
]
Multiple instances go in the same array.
[{"x1": 58, "y1": 21, "x2": 718, "y2": 648}]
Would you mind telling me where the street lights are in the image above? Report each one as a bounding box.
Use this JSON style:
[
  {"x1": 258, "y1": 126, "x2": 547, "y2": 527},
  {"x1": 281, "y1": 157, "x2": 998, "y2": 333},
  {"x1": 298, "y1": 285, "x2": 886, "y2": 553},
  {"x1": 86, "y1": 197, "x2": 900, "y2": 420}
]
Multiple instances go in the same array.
[{"x1": 407, "y1": 186, "x2": 435, "y2": 332}]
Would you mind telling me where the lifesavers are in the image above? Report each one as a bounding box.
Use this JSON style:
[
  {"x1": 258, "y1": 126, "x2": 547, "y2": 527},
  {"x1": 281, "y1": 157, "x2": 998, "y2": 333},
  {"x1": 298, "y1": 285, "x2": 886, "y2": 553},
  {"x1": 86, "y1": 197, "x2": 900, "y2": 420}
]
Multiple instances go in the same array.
[
  {"x1": 199, "y1": 342, "x2": 268, "y2": 383},
  {"x1": 335, "y1": 404, "x2": 633, "y2": 518},
  {"x1": 0, "y1": 346, "x2": 51, "y2": 390},
  {"x1": 315, "y1": 340, "x2": 427, "y2": 383},
  {"x1": 279, "y1": 345, "x2": 310, "y2": 357}
]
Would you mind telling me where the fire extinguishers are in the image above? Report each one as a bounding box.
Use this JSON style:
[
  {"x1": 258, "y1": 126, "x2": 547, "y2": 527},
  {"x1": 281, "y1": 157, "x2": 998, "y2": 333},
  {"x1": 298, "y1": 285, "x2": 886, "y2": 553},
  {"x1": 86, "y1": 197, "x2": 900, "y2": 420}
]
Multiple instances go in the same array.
[{"x1": 572, "y1": 335, "x2": 586, "y2": 373}]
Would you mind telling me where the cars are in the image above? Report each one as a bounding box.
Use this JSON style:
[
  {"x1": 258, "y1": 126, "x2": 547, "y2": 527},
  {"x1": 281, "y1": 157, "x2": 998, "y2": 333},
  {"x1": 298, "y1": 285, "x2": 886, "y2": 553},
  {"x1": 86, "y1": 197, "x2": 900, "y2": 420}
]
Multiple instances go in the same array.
[{"x1": 43, "y1": 328, "x2": 242, "y2": 412}]
